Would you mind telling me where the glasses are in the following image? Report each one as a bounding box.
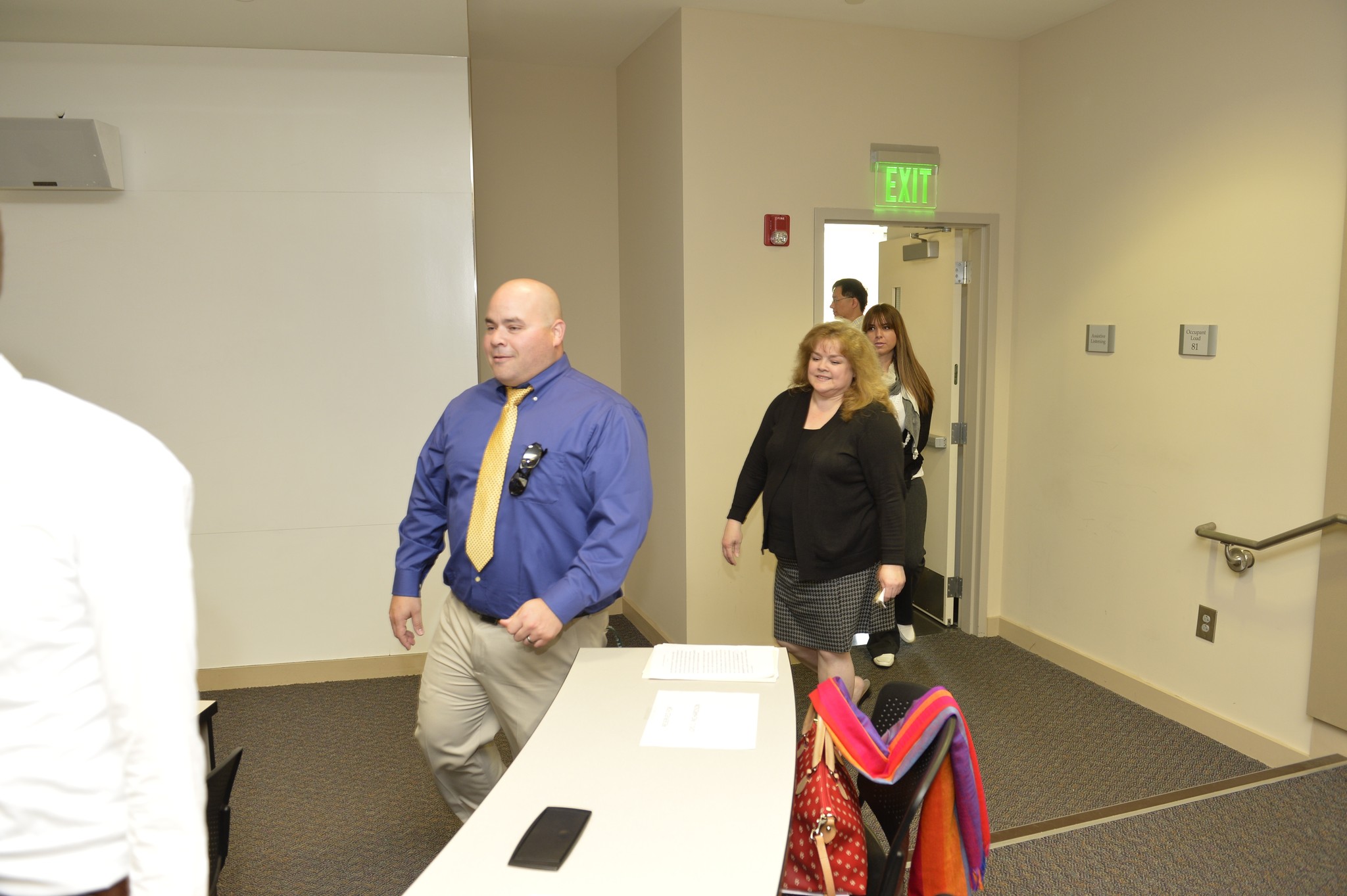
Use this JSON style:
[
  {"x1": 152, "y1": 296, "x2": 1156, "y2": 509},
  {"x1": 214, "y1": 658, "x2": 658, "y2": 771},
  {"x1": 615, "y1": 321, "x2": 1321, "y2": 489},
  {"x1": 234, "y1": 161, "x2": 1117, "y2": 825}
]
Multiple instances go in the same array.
[
  {"x1": 833, "y1": 297, "x2": 854, "y2": 304},
  {"x1": 508, "y1": 442, "x2": 548, "y2": 496}
]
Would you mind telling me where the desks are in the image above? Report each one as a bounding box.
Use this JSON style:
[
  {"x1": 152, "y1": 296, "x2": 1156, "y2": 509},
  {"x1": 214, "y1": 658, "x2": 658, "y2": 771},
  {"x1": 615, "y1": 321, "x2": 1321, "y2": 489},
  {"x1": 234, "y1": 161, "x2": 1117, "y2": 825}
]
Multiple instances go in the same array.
[
  {"x1": 393, "y1": 642, "x2": 798, "y2": 896},
  {"x1": 197, "y1": 697, "x2": 219, "y2": 774}
]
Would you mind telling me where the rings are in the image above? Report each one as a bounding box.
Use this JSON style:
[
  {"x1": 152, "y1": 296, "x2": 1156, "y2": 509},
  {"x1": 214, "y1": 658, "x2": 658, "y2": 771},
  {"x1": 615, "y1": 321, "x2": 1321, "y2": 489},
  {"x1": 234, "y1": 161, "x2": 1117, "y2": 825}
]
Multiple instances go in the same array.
[{"x1": 527, "y1": 637, "x2": 536, "y2": 644}]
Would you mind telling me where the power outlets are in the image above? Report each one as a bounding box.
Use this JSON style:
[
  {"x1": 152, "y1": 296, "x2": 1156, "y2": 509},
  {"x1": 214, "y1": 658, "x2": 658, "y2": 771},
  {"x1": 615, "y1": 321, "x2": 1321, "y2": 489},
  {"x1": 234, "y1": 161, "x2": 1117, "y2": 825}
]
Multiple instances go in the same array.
[{"x1": 1195, "y1": 604, "x2": 1218, "y2": 643}]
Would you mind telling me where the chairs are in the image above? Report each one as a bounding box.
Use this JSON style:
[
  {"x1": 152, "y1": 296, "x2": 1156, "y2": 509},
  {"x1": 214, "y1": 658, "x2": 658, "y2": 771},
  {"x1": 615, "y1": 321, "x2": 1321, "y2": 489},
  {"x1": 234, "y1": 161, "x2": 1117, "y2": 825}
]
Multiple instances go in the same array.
[
  {"x1": 207, "y1": 744, "x2": 244, "y2": 896},
  {"x1": 856, "y1": 677, "x2": 957, "y2": 896}
]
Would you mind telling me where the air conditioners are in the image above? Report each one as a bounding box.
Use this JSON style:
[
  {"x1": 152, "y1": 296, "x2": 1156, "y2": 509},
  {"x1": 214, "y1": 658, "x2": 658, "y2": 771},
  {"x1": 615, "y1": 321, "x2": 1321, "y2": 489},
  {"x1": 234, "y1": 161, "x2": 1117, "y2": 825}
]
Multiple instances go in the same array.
[{"x1": 0, "y1": 116, "x2": 126, "y2": 191}]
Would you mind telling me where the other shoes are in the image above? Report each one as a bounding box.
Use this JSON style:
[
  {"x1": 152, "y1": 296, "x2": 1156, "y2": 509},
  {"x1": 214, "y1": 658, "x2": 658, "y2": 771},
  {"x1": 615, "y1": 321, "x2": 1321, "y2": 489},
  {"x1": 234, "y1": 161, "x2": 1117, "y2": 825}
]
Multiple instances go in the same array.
[
  {"x1": 897, "y1": 623, "x2": 916, "y2": 644},
  {"x1": 873, "y1": 653, "x2": 895, "y2": 668}
]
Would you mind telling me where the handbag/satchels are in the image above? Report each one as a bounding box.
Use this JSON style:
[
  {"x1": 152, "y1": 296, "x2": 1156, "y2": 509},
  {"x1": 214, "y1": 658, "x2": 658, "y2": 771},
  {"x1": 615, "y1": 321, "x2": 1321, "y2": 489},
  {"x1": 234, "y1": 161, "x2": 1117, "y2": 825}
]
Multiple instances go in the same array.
[{"x1": 779, "y1": 708, "x2": 869, "y2": 896}]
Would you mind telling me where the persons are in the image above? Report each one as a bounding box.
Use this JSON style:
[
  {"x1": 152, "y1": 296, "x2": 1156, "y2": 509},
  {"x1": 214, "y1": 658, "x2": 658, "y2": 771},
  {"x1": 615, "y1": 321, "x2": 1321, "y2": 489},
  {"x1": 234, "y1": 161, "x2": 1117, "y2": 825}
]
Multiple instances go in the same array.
[
  {"x1": 390, "y1": 275, "x2": 657, "y2": 824},
  {"x1": 861, "y1": 301, "x2": 935, "y2": 669},
  {"x1": 830, "y1": 279, "x2": 869, "y2": 326},
  {"x1": 2, "y1": 355, "x2": 212, "y2": 896},
  {"x1": 717, "y1": 319, "x2": 912, "y2": 707}
]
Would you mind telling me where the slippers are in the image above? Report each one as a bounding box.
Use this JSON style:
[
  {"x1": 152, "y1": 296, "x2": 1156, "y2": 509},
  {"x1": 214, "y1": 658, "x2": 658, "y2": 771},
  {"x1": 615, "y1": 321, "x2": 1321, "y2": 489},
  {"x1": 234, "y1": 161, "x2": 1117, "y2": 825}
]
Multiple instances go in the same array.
[{"x1": 857, "y1": 679, "x2": 871, "y2": 710}]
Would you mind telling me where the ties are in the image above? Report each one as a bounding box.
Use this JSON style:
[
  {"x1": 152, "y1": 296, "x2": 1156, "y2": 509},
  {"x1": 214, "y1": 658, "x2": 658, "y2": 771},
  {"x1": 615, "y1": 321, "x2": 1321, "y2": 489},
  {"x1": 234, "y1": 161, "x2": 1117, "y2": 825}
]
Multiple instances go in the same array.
[{"x1": 464, "y1": 384, "x2": 534, "y2": 573}]
